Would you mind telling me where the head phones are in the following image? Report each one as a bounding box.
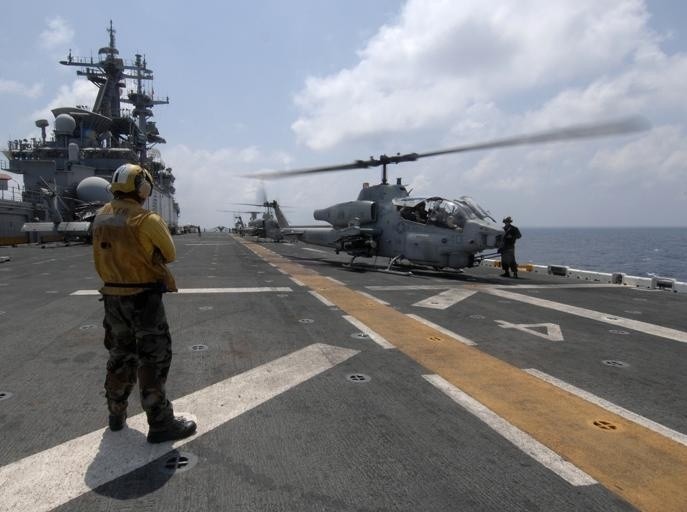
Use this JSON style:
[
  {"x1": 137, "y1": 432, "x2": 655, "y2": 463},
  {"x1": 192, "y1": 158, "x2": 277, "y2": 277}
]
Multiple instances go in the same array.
[{"x1": 137, "y1": 169, "x2": 152, "y2": 199}]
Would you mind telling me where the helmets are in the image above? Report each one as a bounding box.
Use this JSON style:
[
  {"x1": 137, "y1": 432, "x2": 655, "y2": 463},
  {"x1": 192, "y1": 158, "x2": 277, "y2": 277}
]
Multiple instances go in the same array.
[{"x1": 112, "y1": 163, "x2": 155, "y2": 205}]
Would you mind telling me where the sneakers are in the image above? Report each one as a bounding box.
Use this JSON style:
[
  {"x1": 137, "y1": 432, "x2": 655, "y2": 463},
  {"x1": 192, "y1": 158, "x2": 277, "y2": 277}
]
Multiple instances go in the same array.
[
  {"x1": 147, "y1": 419, "x2": 196, "y2": 442},
  {"x1": 500, "y1": 272, "x2": 510, "y2": 276},
  {"x1": 109, "y1": 411, "x2": 127, "y2": 430},
  {"x1": 513, "y1": 271, "x2": 518, "y2": 278}
]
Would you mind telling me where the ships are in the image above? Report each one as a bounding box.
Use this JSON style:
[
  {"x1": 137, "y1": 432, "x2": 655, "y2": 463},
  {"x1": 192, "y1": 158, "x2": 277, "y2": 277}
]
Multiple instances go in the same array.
[{"x1": 0, "y1": 16, "x2": 687, "y2": 512}]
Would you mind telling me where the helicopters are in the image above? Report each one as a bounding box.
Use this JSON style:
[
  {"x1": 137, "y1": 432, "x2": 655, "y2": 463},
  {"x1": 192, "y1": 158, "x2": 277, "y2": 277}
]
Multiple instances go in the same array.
[
  {"x1": 234, "y1": 118, "x2": 651, "y2": 279},
  {"x1": 216, "y1": 198, "x2": 292, "y2": 242}
]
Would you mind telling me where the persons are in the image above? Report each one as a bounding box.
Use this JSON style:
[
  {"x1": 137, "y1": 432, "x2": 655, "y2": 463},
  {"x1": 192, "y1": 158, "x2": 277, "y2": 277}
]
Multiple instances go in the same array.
[
  {"x1": 103, "y1": 129, "x2": 112, "y2": 148},
  {"x1": 90, "y1": 163, "x2": 196, "y2": 443},
  {"x1": 495, "y1": 217, "x2": 522, "y2": 278},
  {"x1": 198, "y1": 226, "x2": 201, "y2": 236}
]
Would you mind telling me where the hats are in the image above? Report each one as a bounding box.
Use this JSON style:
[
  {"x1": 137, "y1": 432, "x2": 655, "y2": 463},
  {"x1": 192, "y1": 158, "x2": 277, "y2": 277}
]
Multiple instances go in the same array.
[{"x1": 503, "y1": 217, "x2": 512, "y2": 223}]
